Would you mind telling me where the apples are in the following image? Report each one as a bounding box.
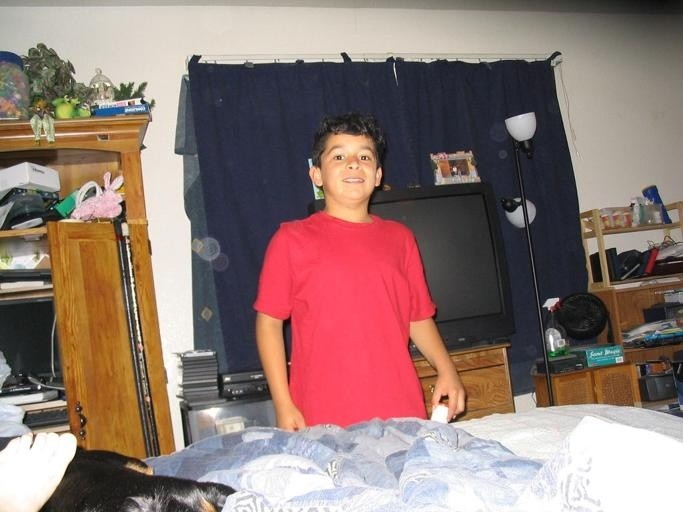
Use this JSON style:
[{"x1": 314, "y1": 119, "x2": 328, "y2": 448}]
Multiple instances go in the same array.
[
  {"x1": 76, "y1": 107, "x2": 91, "y2": 117},
  {"x1": 54, "y1": 102, "x2": 73, "y2": 118}
]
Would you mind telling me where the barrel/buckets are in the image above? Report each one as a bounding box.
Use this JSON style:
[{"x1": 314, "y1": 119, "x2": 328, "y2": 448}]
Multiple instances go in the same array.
[{"x1": 556, "y1": 292, "x2": 610, "y2": 346}]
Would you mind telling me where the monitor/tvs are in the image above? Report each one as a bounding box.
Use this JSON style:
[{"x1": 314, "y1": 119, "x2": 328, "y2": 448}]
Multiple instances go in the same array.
[
  {"x1": 307, "y1": 180, "x2": 517, "y2": 359},
  {"x1": 0, "y1": 296, "x2": 62, "y2": 383}
]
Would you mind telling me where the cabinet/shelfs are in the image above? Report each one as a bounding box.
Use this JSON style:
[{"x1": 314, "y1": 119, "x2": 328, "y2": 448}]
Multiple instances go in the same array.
[
  {"x1": 412, "y1": 342, "x2": 515, "y2": 424},
  {"x1": 0, "y1": 106, "x2": 178, "y2": 461},
  {"x1": 534, "y1": 200, "x2": 682, "y2": 418}
]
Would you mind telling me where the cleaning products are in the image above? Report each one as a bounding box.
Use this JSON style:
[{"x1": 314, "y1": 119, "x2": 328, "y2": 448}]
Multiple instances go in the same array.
[{"x1": 543, "y1": 297, "x2": 568, "y2": 358}]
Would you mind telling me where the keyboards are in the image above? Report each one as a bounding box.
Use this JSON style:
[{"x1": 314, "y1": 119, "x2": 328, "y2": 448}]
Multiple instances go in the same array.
[{"x1": 23, "y1": 407, "x2": 68, "y2": 427}]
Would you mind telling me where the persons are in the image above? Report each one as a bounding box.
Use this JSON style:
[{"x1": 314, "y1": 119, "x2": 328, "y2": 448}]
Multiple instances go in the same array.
[
  {"x1": 0, "y1": 431, "x2": 77, "y2": 512},
  {"x1": 253, "y1": 113, "x2": 466, "y2": 431}
]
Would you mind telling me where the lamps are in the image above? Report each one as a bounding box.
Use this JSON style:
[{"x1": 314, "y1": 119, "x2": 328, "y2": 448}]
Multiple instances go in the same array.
[{"x1": 500, "y1": 112, "x2": 561, "y2": 406}]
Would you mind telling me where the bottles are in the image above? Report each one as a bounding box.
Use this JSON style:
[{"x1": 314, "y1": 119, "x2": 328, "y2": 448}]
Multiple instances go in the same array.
[
  {"x1": 0, "y1": 51, "x2": 31, "y2": 118},
  {"x1": 89, "y1": 67, "x2": 114, "y2": 99}
]
